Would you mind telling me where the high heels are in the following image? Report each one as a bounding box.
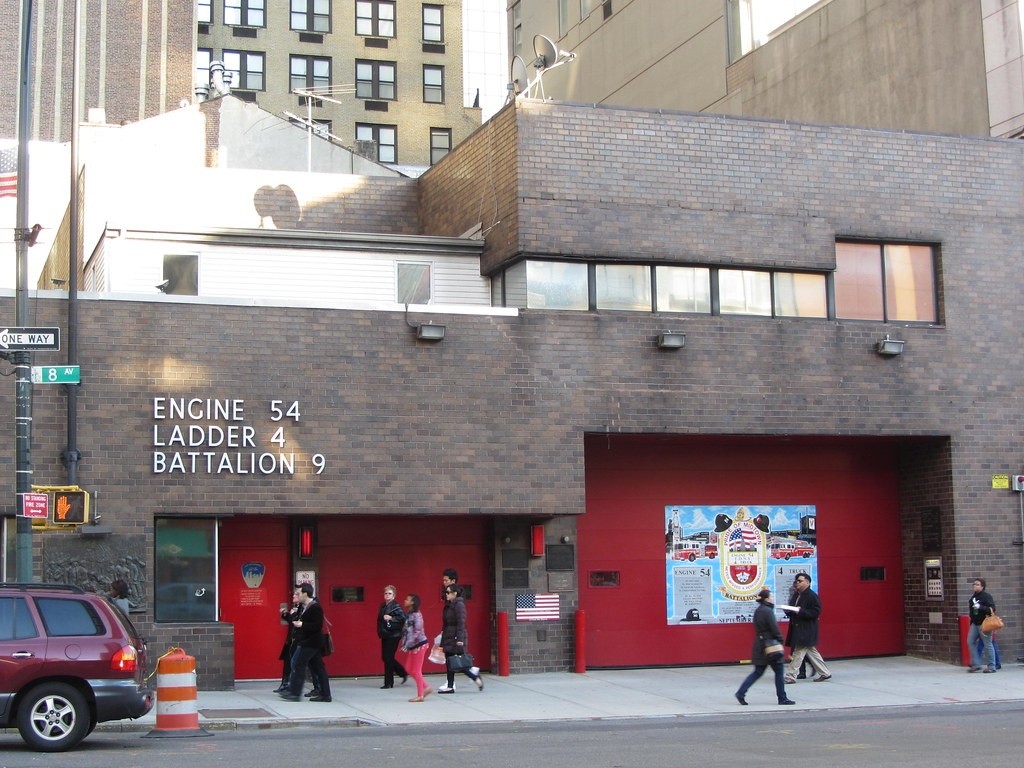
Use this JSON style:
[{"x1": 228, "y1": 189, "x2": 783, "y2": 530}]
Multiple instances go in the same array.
[
  {"x1": 400, "y1": 671, "x2": 410, "y2": 684},
  {"x1": 382, "y1": 681, "x2": 394, "y2": 688}
]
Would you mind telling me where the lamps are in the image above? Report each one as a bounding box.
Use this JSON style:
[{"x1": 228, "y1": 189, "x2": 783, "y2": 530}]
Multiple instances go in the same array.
[
  {"x1": 877, "y1": 339, "x2": 905, "y2": 355},
  {"x1": 658, "y1": 333, "x2": 686, "y2": 349},
  {"x1": 416, "y1": 324, "x2": 446, "y2": 341}
]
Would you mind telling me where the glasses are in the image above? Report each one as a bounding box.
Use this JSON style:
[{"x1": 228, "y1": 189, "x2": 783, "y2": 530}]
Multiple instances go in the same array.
[
  {"x1": 445, "y1": 590, "x2": 454, "y2": 595},
  {"x1": 797, "y1": 580, "x2": 807, "y2": 584},
  {"x1": 972, "y1": 583, "x2": 982, "y2": 586}
]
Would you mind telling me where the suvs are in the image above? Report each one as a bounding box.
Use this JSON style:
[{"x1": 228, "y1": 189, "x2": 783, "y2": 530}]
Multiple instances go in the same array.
[{"x1": 0, "y1": 583, "x2": 155, "y2": 753}]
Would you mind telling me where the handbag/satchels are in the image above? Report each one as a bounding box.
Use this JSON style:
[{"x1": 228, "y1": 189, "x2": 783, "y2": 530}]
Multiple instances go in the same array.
[
  {"x1": 385, "y1": 607, "x2": 405, "y2": 632},
  {"x1": 447, "y1": 642, "x2": 472, "y2": 672},
  {"x1": 982, "y1": 607, "x2": 1003, "y2": 633},
  {"x1": 760, "y1": 638, "x2": 784, "y2": 660},
  {"x1": 428, "y1": 632, "x2": 446, "y2": 665},
  {"x1": 320, "y1": 633, "x2": 333, "y2": 655}
]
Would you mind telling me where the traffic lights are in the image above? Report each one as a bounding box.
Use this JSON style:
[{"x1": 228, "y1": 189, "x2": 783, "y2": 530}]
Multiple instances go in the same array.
[{"x1": 52, "y1": 490, "x2": 89, "y2": 525}]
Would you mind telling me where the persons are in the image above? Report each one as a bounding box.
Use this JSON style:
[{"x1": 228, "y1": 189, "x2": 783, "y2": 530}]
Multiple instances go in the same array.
[
  {"x1": 968, "y1": 610, "x2": 1002, "y2": 669},
  {"x1": 735, "y1": 590, "x2": 797, "y2": 705},
  {"x1": 783, "y1": 573, "x2": 833, "y2": 684},
  {"x1": 437, "y1": 569, "x2": 483, "y2": 694},
  {"x1": 376, "y1": 584, "x2": 408, "y2": 689},
  {"x1": 401, "y1": 594, "x2": 432, "y2": 702},
  {"x1": 967, "y1": 577, "x2": 997, "y2": 673},
  {"x1": 111, "y1": 581, "x2": 130, "y2": 616},
  {"x1": 271, "y1": 583, "x2": 331, "y2": 702}
]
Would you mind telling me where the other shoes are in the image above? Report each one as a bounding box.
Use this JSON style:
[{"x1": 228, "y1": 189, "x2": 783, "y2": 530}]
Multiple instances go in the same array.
[
  {"x1": 782, "y1": 668, "x2": 832, "y2": 683},
  {"x1": 470, "y1": 666, "x2": 479, "y2": 676},
  {"x1": 270, "y1": 684, "x2": 333, "y2": 702},
  {"x1": 439, "y1": 679, "x2": 459, "y2": 693},
  {"x1": 409, "y1": 697, "x2": 424, "y2": 702},
  {"x1": 475, "y1": 674, "x2": 484, "y2": 691},
  {"x1": 736, "y1": 691, "x2": 748, "y2": 705},
  {"x1": 423, "y1": 685, "x2": 432, "y2": 697},
  {"x1": 966, "y1": 665, "x2": 1001, "y2": 675},
  {"x1": 779, "y1": 698, "x2": 794, "y2": 704},
  {"x1": 438, "y1": 687, "x2": 456, "y2": 693}
]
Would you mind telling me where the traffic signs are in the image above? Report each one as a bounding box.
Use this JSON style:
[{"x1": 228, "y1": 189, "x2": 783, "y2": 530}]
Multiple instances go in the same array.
[
  {"x1": 23, "y1": 492, "x2": 48, "y2": 518},
  {"x1": 0, "y1": 327, "x2": 61, "y2": 352}
]
